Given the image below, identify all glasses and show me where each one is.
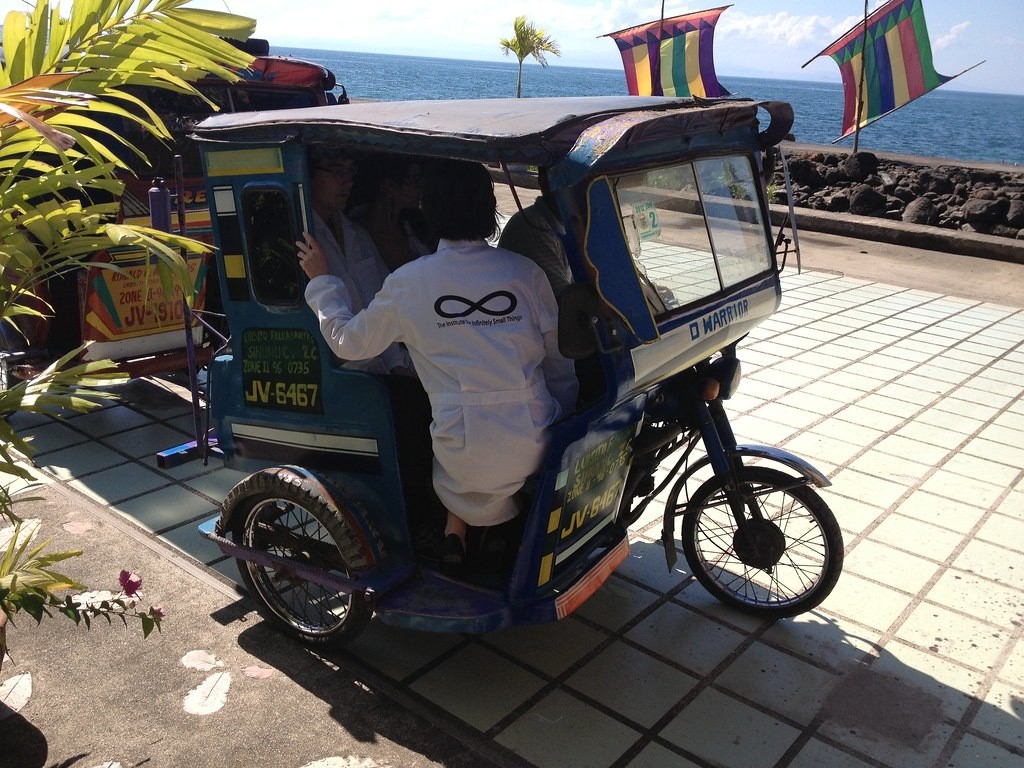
[315,157,360,177]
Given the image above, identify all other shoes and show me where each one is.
[411,521,446,563]
[442,533,471,577]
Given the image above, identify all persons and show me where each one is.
[298,149,612,567]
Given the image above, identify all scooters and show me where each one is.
[151,98,846,654]
[1,35,352,412]
